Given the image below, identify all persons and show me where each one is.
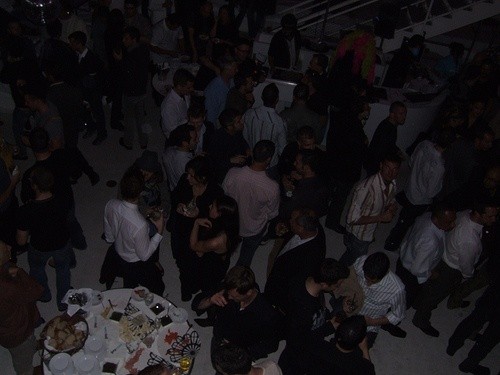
[0,0,500,375]
[191,266,280,375]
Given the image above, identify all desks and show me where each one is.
[43,288,195,375]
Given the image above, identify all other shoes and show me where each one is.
[83,127,96,139]
[447,298,470,308]
[89,171,99,186]
[93,132,106,146]
[446,339,464,355]
[120,137,132,149]
[459,363,490,375]
[413,319,439,337]
[326,221,346,234]
[382,322,406,337]
[114,123,125,131]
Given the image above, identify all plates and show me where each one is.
[42,315,88,356]
